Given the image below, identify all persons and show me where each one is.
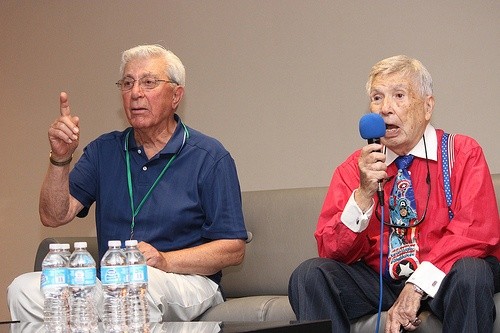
[287,54,500,333]
[5,44,249,322]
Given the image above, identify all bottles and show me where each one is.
[39,244,68,333]
[100,240,127,333]
[61,244,72,260]
[123,239,150,333]
[67,242,97,333]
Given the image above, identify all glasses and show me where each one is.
[116,77,179,90]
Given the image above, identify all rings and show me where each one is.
[411,317,422,327]
[403,322,411,328]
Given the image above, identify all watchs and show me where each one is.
[49,150,73,166]
[413,284,425,296]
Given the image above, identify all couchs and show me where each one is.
[33,173,500,333]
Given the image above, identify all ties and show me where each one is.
[386,155,420,282]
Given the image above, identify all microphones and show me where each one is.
[359,112,386,206]
